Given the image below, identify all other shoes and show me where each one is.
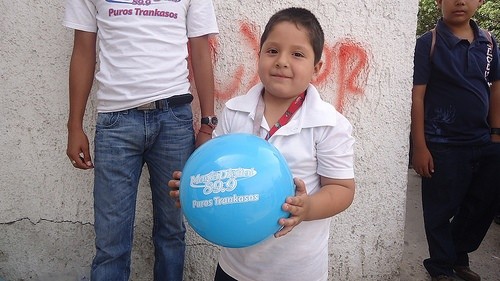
[431,267,481,281]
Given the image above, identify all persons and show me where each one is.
[62,0,220,281]
[411,0,500,281]
[169,7,355,281]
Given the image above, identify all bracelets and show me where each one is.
[199,128,213,134]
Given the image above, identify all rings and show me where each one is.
[71,161,76,164]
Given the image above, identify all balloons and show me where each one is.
[180,133,295,249]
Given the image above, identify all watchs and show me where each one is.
[200,117,218,127]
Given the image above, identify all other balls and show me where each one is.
[177,130,297,248]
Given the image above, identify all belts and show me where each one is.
[136,94,193,111]
